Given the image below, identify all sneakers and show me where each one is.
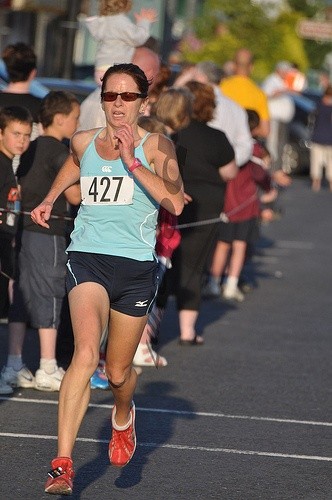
[1,364,35,388]
[107,399,137,467]
[33,367,66,391]
[133,343,168,366]
[44,457,75,496]
[90,367,109,390]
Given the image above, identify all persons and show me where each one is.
[1,0,332,398]
[28,64,190,495]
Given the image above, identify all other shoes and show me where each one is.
[201,285,222,299]
[223,287,244,302]
[0,378,14,393]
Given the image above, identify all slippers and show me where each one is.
[178,334,204,345]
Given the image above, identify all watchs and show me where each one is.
[128,158,141,172]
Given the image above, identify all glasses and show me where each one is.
[102,92,146,101]
